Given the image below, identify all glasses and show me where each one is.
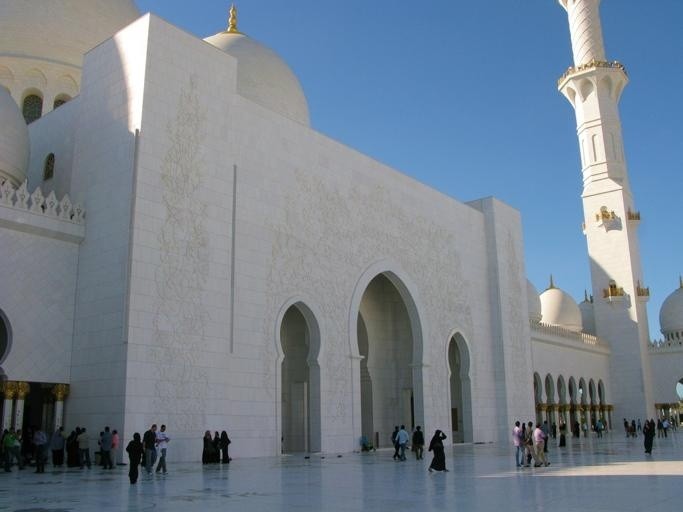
[162,428,166,430]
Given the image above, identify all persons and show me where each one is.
[111,430,119,467]
[552,422,556,437]
[572,419,609,439]
[512,421,525,468]
[524,421,542,468]
[202,429,232,465]
[427,429,450,473]
[533,423,551,467]
[522,423,526,437]
[622,418,641,439]
[392,426,401,460]
[142,424,157,475]
[643,420,655,455]
[97,432,104,447]
[542,421,549,452]
[412,425,425,460]
[101,426,113,470]
[0,425,92,475]
[126,432,144,484]
[394,425,410,461]
[559,419,567,447]
[155,424,170,475]
[656,416,676,439]
